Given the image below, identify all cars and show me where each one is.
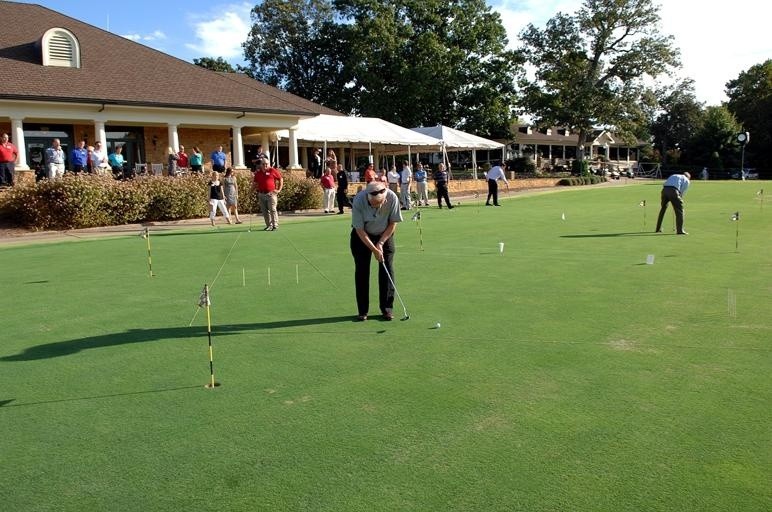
[725,166,760,181]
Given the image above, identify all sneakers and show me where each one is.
[264,225,279,232]
[211,219,244,228]
[485,203,503,207]
[323,208,344,215]
[400,202,454,210]
[676,230,689,235]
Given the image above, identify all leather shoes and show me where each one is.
[383,312,395,321]
[358,314,368,321]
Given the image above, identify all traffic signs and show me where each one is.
[601,142,610,177]
[578,142,586,178]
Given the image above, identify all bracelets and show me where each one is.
[379,241,384,245]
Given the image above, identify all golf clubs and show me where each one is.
[382,260,410,321]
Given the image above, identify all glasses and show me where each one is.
[367,188,386,196]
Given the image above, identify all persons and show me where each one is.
[0,132,20,187]
[702,167,707,180]
[44,136,284,231]
[486,162,509,206]
[655,171,691,234]
[350,182,404,321]
[311,144,454,214]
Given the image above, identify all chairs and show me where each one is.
[134,163,147,173]
[151,163,164,176]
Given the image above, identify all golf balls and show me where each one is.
[436,323,441,327]
